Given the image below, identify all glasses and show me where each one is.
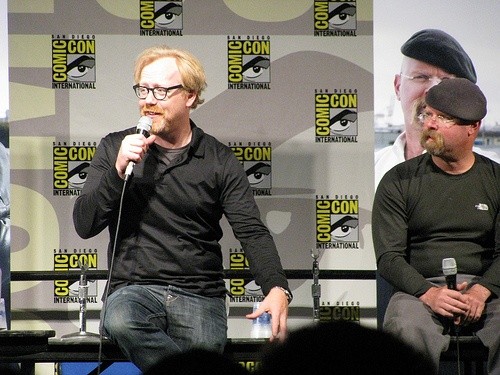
[418,111,459,128]
[133,83,187,100]
[400,72,455,88]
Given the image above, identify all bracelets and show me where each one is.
[275,287,293,303]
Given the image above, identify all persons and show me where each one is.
[72,46,293,373]
[372,77,500,375]
[138,320,437,375]
[371,30,500,192]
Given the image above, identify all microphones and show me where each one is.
[125,116,153,182]
[442,257,460,333]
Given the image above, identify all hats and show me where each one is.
[400,29,477,84]
[424,78,487,122]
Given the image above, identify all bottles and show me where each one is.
[251,303,271,338]
